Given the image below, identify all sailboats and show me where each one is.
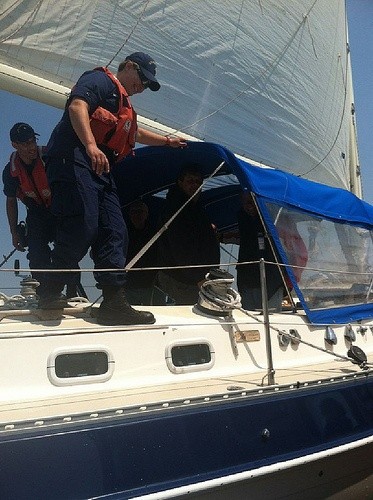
[1,1,373,498]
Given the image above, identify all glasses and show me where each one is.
[11,136,39,147]
[137,69,149,89]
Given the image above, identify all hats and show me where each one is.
[10,122,40,140]
[126,52,160,91]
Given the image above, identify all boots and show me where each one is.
[38,276,67,309]
[67,279,80,303]
[96,287,155,324]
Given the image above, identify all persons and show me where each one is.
[211,192,308,312]
[123,200,163,305]
[157,162,221,306]
[3,124,79,300]
[36,52,187,325]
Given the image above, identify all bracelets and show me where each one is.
[165,136,171,145]
